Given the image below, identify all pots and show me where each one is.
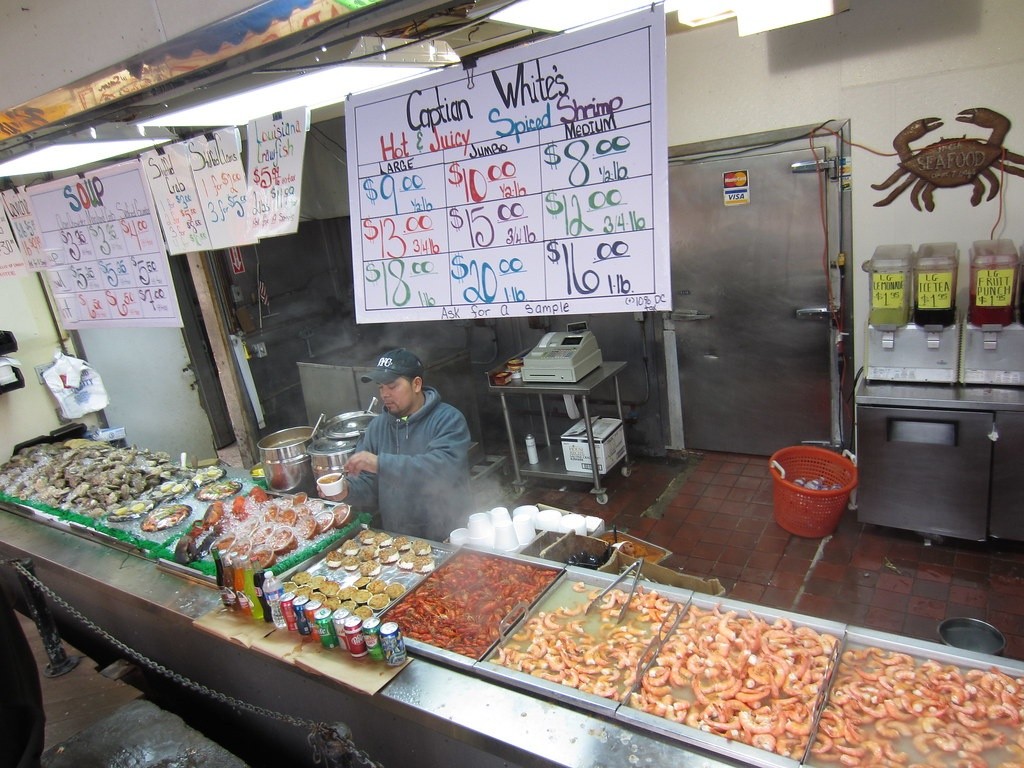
[307,437,359,485]
[257,425,323,498]
[323,410,379,439]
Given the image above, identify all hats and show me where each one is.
[360,348,424,385]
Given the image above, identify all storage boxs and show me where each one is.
[562,414,625,475]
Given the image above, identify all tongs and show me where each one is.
[584,557,644,625]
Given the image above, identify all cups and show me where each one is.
[450,504,587,554]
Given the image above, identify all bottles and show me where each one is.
[249,556,274,622]
[218,549,242,611]
[211,545,231,607]
[261,570,287,628]
[238,554,264,618]
[229,552,251,613]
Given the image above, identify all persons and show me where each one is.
[317,344,473,542]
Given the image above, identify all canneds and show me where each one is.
[305,599,323,641]
[314,607,339,649]
[292,594,311,637]
[333,607,351,650]
[379,622,407,667]
[344,616,368,658]
[362,617,386,661]
[279,592,298,631]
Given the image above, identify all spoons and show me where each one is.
[365,395,378,416]
[337,471,345,482]
[308,412,326,440]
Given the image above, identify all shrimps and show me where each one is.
[487,579,1023,768]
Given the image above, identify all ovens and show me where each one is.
[352,346,487,467]
[296,344,393,431]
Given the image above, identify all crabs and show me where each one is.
[871,107,1024,212]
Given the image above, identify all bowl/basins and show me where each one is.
[316,473,344,496]
